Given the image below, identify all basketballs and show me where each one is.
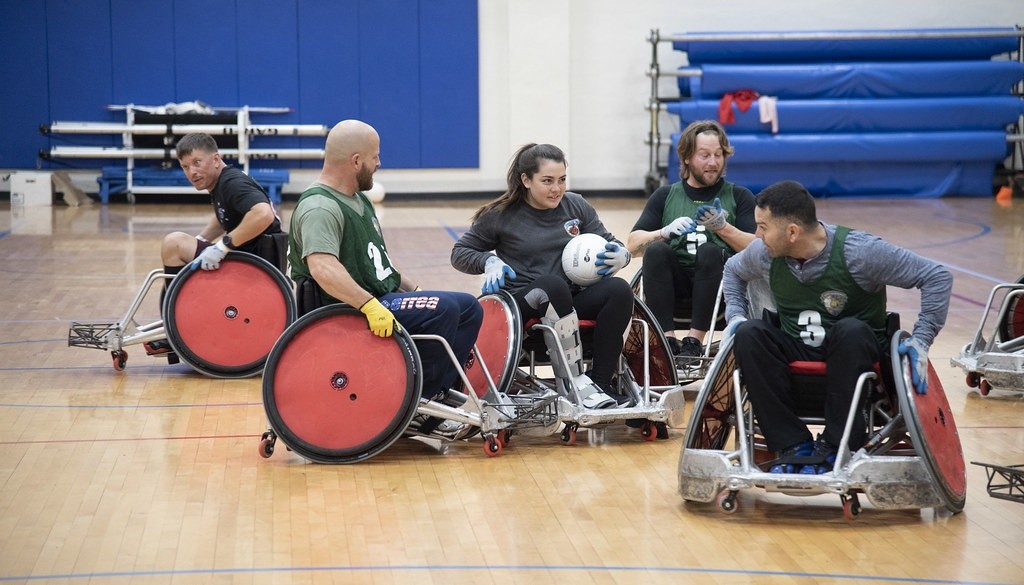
[561,233,612,286]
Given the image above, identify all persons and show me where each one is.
[627,121,756,367]
[723,180,953,472]
[451,144,633,409]
[289,120,483,433]
[142,132,282,355]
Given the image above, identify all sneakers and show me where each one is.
[674,339,702,369]
[766,444,812,472]
[668,336,681,358]
[799,445,835,475]
[409,411,467,436]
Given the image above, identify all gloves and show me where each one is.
[691,198,726,231]
[190,241,225,271]
[898,334,929,394]
[360,299,403,337]
[415,286,423,293]
[597,241,629,278]
[718,311,749,350]
[480,256,517,295]
[661,218,698,241]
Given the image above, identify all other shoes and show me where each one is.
[146,339,173,354]
[598,382,633,409]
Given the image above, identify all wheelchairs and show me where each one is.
[402,288,687,444]
[621,266,728,387]
[675,310,968,522]
[68,228,298,381]
[949,272,1023,397]
[257,274,563,465]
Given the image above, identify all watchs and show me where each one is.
[223,235,237,250]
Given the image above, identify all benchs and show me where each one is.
[97,168,289,205]
